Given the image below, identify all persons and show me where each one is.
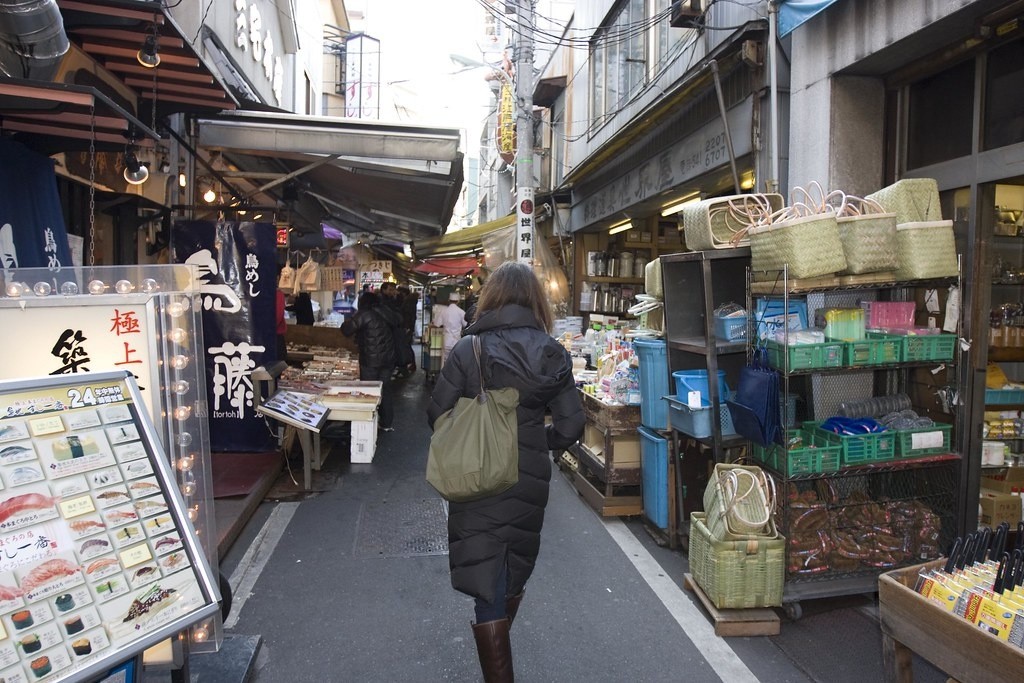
[276,285,288,361]
[424,262,587,683]
[340,282,418,433]
[295,290,314,326]
[294,291,315,326]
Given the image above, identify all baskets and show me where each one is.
[631,258,668,339]
[684,175,961,282]
[688,463,786,610]
[752,330,958,472]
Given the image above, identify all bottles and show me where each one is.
[621,252,633,278]
[604,289,611,311]
[607,255,619,277]
[636,259,648,278]
[629,300,634,308]
[1011,487,1024,522]
[611,294,619,311]
[596,252,606,276]
[988,304,1024,347]
[619,299,625,312]
[593,286,600,311]
[977,503,983,529]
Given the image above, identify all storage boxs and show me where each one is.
[424,327,442,371]
[623,230,652,244]
[657,227,684,247]
[289,380,383,411]
[982,441,1005,465]
[757,301,957,370]
[630,338,668,529]
[584,424,640,469]
[979,467,1024,532]
[350,415,378,463]
[985,389,1024,405]
[714,315,748,343]
[753,418,953,478]
[660,394,800,439]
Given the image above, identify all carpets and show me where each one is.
[186,452,279,497]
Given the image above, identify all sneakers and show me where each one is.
[377,421,394,431]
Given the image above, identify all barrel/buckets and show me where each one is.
[672,369,730,407]
[634,338,668,429]
[637,426,668,528]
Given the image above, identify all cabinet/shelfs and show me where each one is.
[659,246,809,549]
[570,231,654,331]
[744,241,968,620]
[553,388,642,519]
[878,552,1024,682]
[957,234,1023,441]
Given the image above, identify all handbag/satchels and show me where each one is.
[426,387,523,503]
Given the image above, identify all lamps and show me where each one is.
[661,193,706,217]
[252,212,263,220]
[124,153,148,185]
[608,219,637,235]
[137,38,160,66]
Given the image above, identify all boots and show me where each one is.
[470,587,523,683]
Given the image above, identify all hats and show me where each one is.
[449,292,459,301]
